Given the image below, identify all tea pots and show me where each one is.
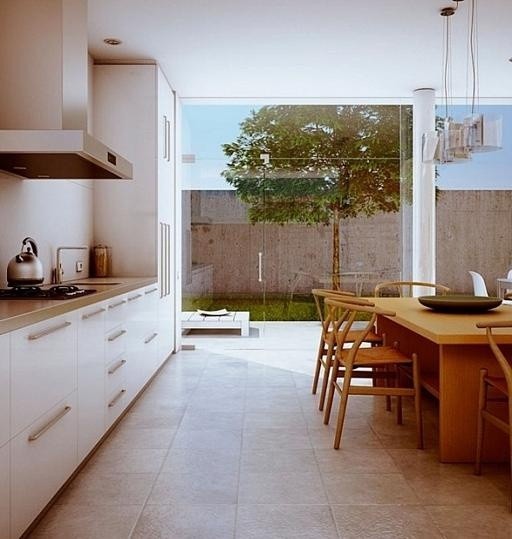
[6,235,45,290]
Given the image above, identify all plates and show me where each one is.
[417,294,503,310]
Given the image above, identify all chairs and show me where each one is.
[283,266,403,318]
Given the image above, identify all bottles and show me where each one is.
[92,244,111,278]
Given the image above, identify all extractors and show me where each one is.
[2,0,134,182]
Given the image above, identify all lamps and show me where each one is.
[420,0,504,166]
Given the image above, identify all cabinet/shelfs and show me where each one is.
[91,64,183,364]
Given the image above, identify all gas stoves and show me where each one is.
[0,286,98,301]
